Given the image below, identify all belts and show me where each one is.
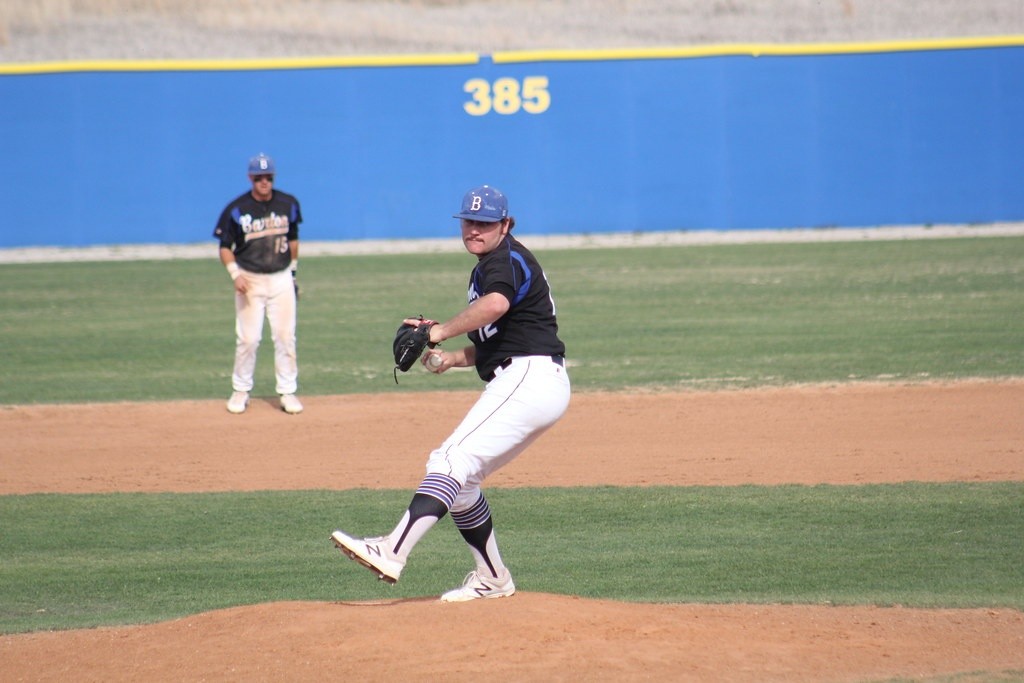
[490,355,566,380]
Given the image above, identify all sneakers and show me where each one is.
[441,568,515,602]
[330,532,404,587]
[227,390,249,414]
[279,393,303,414]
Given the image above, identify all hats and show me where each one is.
[453,185,509,223]
[248,154,275,175]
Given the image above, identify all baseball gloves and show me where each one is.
[393,315,442,384]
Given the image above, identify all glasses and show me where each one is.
[252,177,273,182]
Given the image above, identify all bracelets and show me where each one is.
[291,259,298,271]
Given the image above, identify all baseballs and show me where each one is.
[425,354,442,372]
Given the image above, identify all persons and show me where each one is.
[328,185,571,604]
[212,155,304,415]
